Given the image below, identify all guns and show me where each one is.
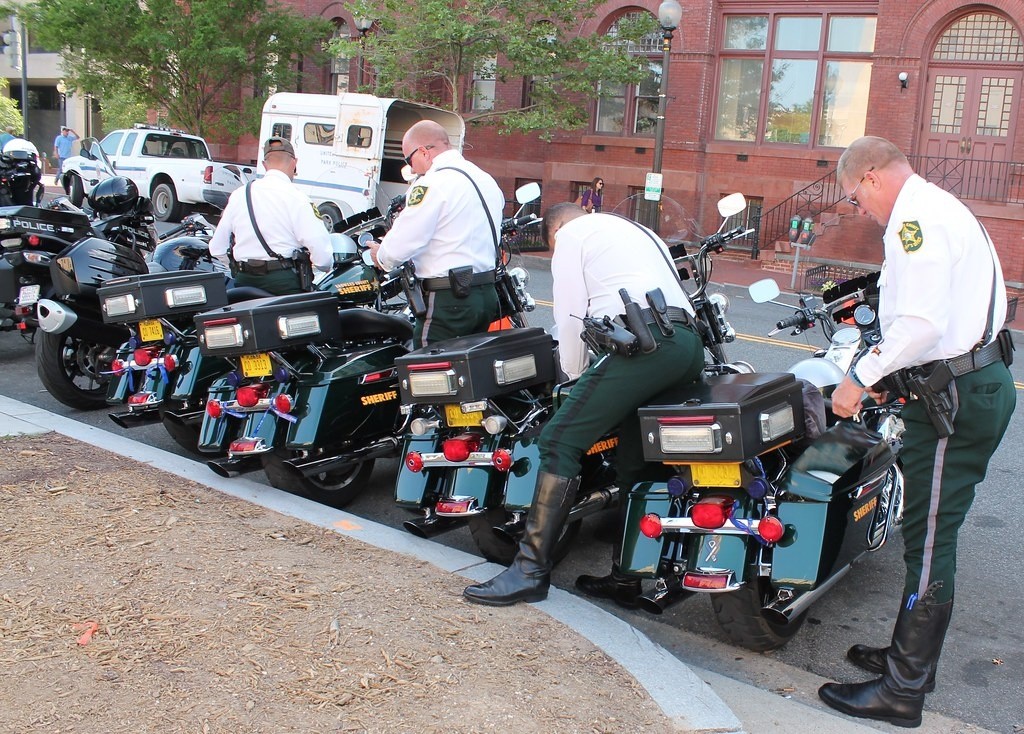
[292,250,313,291]
[906,362,956,438]
[401,260,416,283]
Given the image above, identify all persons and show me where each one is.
[461,202,705,606]
[0,126,16,153]
[581,178,604,215]
[54,126,80,186]
[817,136,1016,728]
[366,119,506,352]
[208,137,334,295]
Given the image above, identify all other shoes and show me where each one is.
[54,177,59,185]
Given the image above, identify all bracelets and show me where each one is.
[849,366,865,388]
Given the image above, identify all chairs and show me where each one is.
[170,147,184,157]
[142,145,147,154]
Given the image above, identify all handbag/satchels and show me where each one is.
[493,270,523,321]
[575,189,593,213]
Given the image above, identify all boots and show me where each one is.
[818,591,953,728]
[464,471,582,607]
[576,493,644,610]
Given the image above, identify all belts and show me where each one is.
[423,271,498,288]
[240,259,292,271]
[908,340,1002,375]
[643,306,699,330]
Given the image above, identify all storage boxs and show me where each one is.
[394,326,556,404]
[48,237,149,298]
[192,291,343,356]
[96,270,228,325]
[286,344,408,449]
[0,205,96,243]
[638,372,806,462]
[772,422,896,589]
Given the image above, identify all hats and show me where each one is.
[263,137,295,157]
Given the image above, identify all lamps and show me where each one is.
[899,71,909,93]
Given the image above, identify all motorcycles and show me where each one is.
[0,134,909,653]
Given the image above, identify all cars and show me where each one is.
[0,133,42,204]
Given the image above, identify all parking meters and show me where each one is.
[786,215,812,290]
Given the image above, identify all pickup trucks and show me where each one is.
[61,122,256,224]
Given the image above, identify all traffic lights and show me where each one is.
[1,16,23,72]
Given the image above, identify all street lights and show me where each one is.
[646,1,684,226]
[56,79,70,128]
[352,8,375,91]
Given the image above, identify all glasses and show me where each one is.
[597,182,603,185]
[404,145,434,167]
[845,166,877,206]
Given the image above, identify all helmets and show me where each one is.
[88,177,139,212]
[786,358,847,400]
